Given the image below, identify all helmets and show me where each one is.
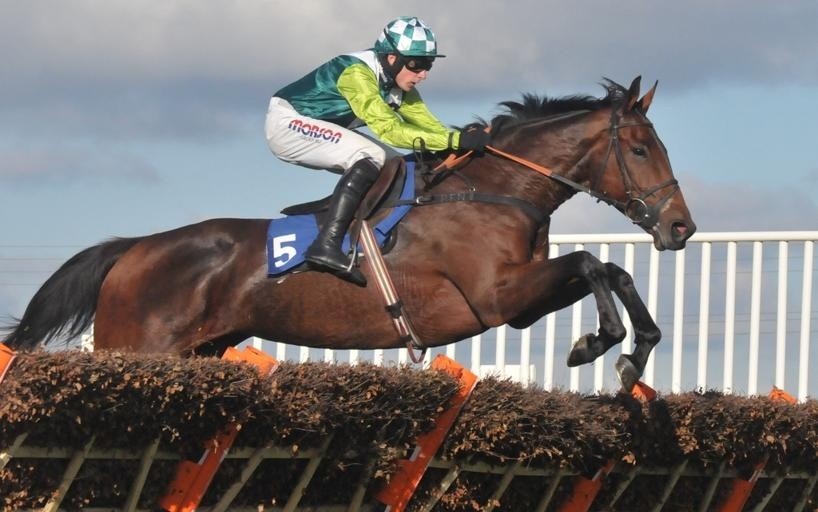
[374,17,447,58]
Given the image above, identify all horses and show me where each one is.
[1,74,697,396]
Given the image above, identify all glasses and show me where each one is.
[402,56,433,72]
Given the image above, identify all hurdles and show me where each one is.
[0,344,813,511]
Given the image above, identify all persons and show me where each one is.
[266,16,492,286]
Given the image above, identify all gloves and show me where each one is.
[459,131,489,154]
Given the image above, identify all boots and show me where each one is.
[304,159,380,286]
